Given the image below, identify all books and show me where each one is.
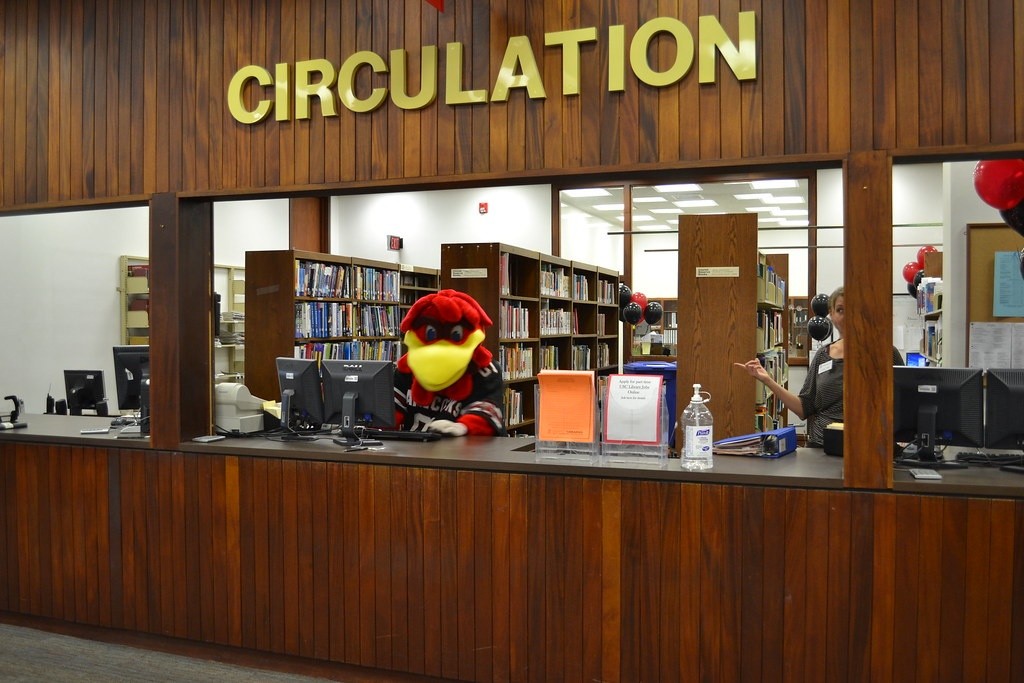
[215,309,245,378]
[755,261,788,436]
[541,262,588,301]
[295,258,438,363]
[598,313,606,336]
[499,299,570,340]
[574,309,579,335]
[505,375,609,442]
[916,276,943,367]
[633,312,678,354]
[599,280,615,305]
[500,251,511,296]
[711,427,797,457]
[498,342,610,381]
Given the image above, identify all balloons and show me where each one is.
[807,293,832,341]
[619,283,663,329]
[973,158,1024,281]
[902,245,938,299]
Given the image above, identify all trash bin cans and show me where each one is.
[624,361,678,450]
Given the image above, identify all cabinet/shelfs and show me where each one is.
[788,295,809,367]
[632,298,677,355]
[120,252,246,387]
[679,211,790,435]
[918,309,943,363]
[441,241,620,435]
[245,249,439,397]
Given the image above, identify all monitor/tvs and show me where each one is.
[63,368,105,409]
[275,357,324,441]
[112,344,150,438]
[892,366,984,470]
[985,368,1024,474]
[321,359,395,446]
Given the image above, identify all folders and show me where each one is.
[712,428,797,458]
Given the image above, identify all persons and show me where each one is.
[733,287,905,448]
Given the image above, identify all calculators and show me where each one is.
[909,468,943,479]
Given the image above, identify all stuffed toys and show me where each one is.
[389,288,504,438]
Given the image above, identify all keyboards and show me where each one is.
[338,427,441,442]
[955,452,1024,466]
